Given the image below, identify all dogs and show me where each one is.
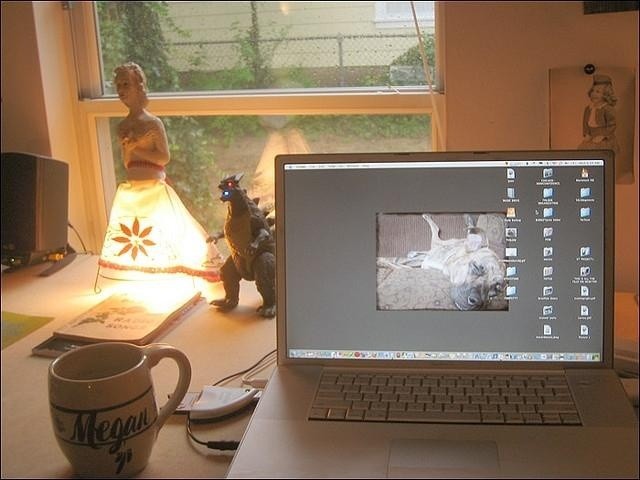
[376,213,507,310]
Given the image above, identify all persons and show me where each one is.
[578,73,618,153]
[111,61,171,181]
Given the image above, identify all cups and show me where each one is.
[48,341,192,480]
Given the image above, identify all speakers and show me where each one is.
[0,150,68,268]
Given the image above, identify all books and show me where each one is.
[31,295,208,360]
[53,286,203,347]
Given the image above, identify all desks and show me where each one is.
[1,247,640,479]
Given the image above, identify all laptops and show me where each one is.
[227,150,639,479]
